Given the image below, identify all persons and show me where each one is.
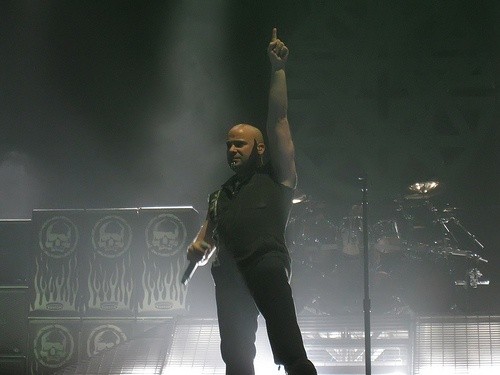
[186,28,317,375]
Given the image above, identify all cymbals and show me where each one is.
[402,181,446,200]
[435,202,462,210]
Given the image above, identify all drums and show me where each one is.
[372,220,405,254]
[339,215,370,256]
[285,216,336,252]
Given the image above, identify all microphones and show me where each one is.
[181,242,208,285]
